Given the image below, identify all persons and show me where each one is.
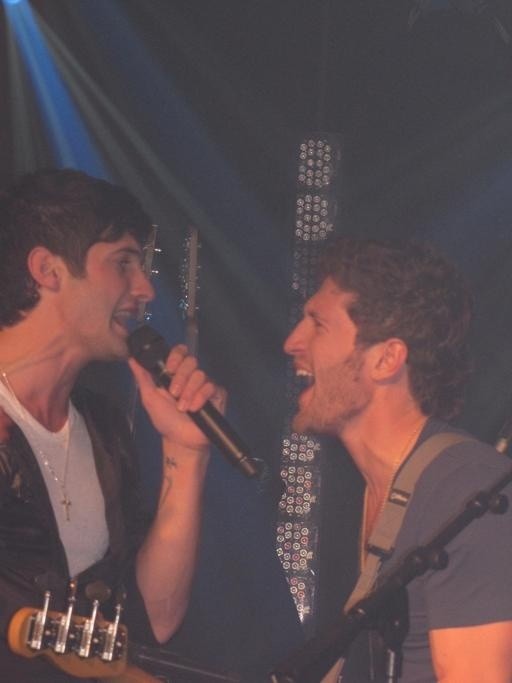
[283,235,512,683]
[0,165,229,683]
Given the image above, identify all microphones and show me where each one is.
[126,323,262,481]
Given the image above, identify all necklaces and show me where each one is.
[361,416,427,574]
[0,368,72,521]
